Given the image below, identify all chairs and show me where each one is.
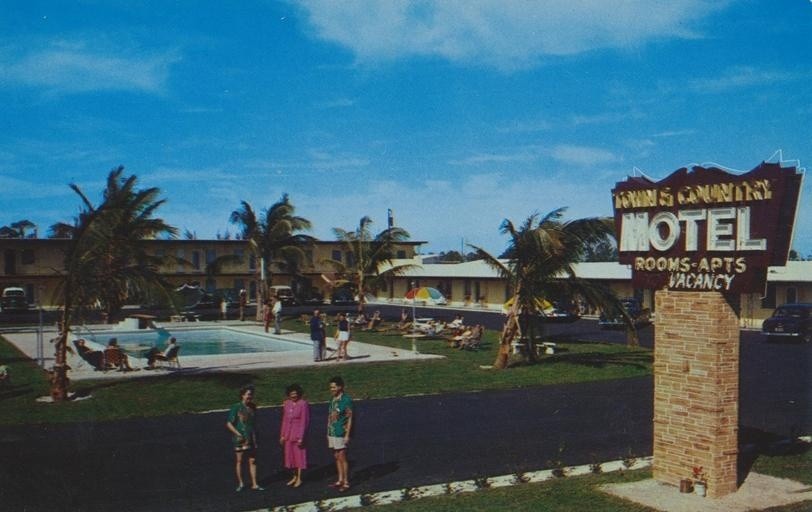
[297,307,487,353]
[68,335,182,378]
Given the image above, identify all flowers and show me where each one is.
[688,464,706,482]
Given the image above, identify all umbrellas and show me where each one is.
[504,293,551,313]
[403,286,445,305]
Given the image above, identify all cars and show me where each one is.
[760,302,811,343]
[331,288,355,305]
[299,287,323,305]
[544,303,580,322]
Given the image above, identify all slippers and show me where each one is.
[235,483,246,494]
[251,485,267,492]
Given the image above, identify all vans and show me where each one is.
[269,285,296,307]
[214,288,241,309]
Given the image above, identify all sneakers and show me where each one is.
[327,481,343,489]
[337,483,354,493]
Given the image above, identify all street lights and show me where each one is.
[409,280,418,353]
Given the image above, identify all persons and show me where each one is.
[271,295,283,334]
[145,336,176,368]
[279,384,310,488]
[307,308,484,361]
[220,298,227,319]
[326,378,353,491]
[262,299,271,333]
[109,337,133,369]
[240,290,246,322]
[227,385,266,493]
[77,337,106,367]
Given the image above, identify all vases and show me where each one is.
[693,481,706,496]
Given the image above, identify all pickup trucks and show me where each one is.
[0,287,29,313]
[599,297,649,331]
